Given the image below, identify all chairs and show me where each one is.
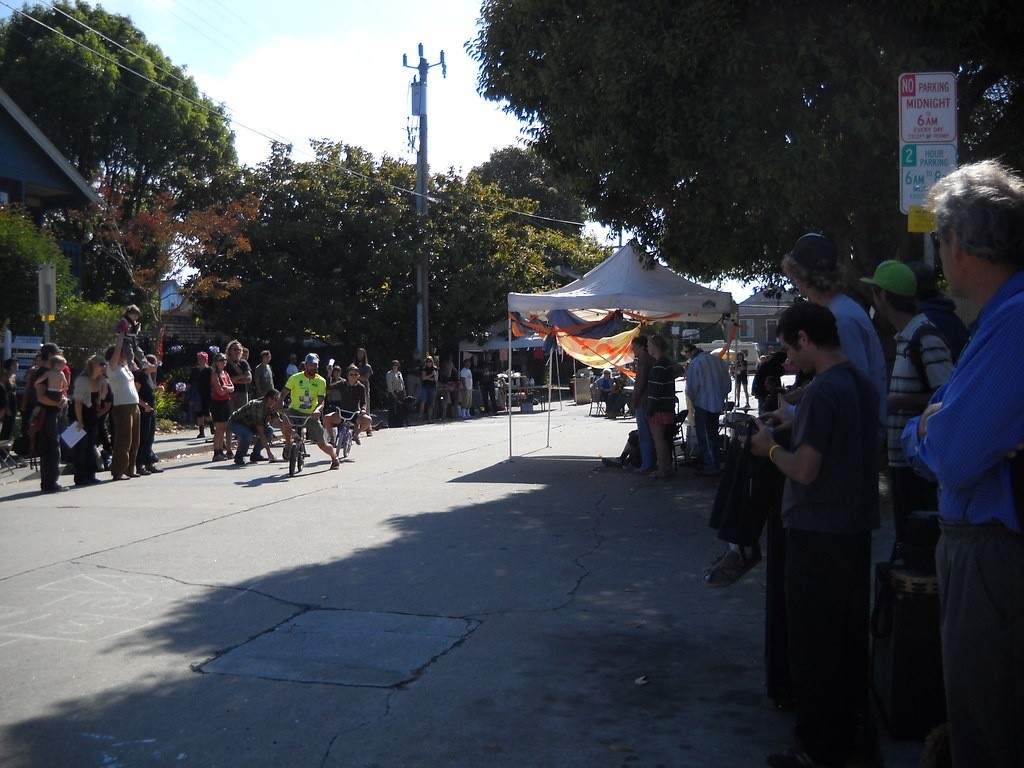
[0,421,18,475]
[718,402,735,435]
[589,385,606,416]
[670,410,690,469]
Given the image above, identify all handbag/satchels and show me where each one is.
[900,510,941,576]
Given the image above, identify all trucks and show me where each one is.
[697,340,759,373]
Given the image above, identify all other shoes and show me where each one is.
[331,459,340,470]
[282,444,292,460]
[634,468,654,474]
[328,439,334,444]
[649,469,673,479]
[702,541,762,587]
[601,458,623,469]
[129,364,156,371]
[353,436,361,445]
[196,431,268,464]
[695,470,720,476]
[41,466,164,490]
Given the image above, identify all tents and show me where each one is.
[458,317,561,404]
[506,239,739,464]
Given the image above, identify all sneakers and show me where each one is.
[767,744,830,768]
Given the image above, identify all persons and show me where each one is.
[592,233,975,586]
[899,158,1024,767]
[0,305,498,492]
[737,300,886,767]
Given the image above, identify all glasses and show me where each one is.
[929,231,945,249]
[350,373,358,377]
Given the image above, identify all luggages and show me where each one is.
[870,563,948,740]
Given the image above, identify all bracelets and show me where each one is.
[769,445,782,463]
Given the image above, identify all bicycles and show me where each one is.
[329,404,367,459]
[274,408,319,477]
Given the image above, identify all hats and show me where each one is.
[90,354,107,364]
[305,353,320,364]
[791,233,839,269]
[41,343,64,355]
[859,260,917,296]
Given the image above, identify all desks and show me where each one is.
[622,386,635,417]
[502,384,552,413]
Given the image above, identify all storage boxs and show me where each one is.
[520,399,533,413]
